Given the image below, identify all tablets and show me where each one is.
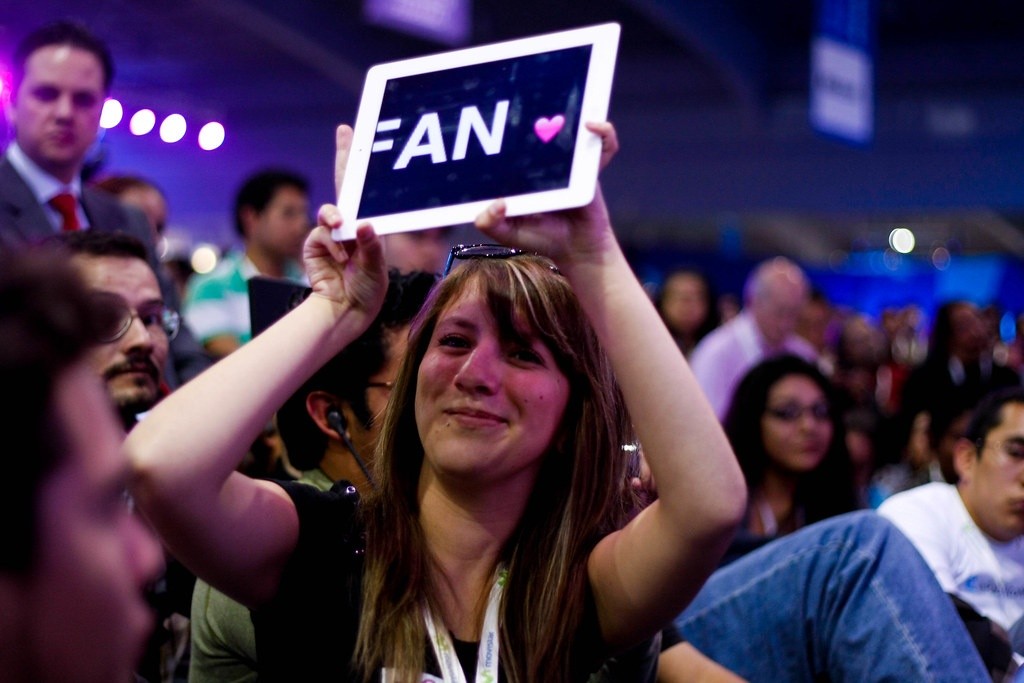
[328,23,620,241]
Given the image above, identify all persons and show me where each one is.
[113,123,752,683]
[637,253,1024,683]
[0,20,178,391]
[0,168,446,683]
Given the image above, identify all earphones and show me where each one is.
[326,407,347,434]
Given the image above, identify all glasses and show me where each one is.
[96,303,179,343]
[442,244,562,275]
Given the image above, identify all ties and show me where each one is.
[48,194,81,233]
